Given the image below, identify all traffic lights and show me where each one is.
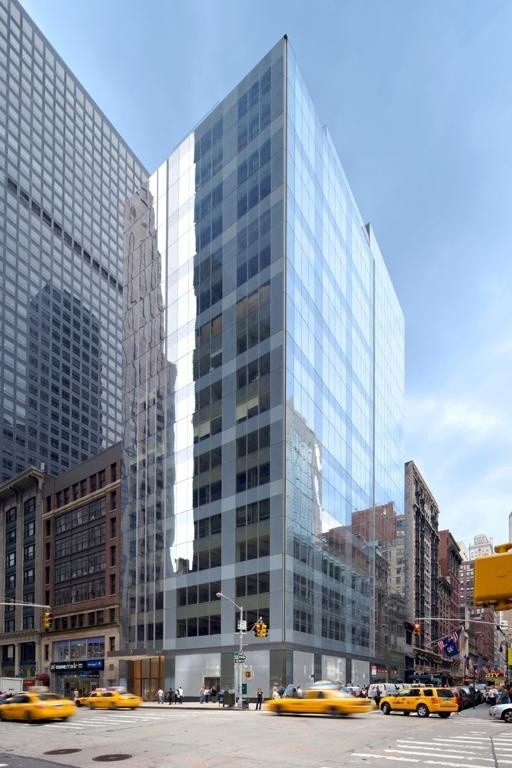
[254,624,260,636]
[414,624,419,637]
[262,624,268,638]
[45,612,53,628]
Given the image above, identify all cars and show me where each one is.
[489,704,512,722]
[262,680,376,718]
[86,687,142,710]
[76,687,108,707]
[1,691,21,700]
[448,683,497,710]
[1,686,77,723]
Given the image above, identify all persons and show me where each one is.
[72,688,80,697]
[154,684,381,710]
[488,684,499,706]
[499,685,509,704]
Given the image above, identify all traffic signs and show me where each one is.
[235,658,245,663]
[239,655,247,658]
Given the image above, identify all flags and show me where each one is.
[436,627,463,657]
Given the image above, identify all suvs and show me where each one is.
[379,687,459,717]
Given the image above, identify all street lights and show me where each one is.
[499,640,508,679]
[216,592,244,709]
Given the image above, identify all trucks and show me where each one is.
[0,676,23,693]
[368,682,433,704]
